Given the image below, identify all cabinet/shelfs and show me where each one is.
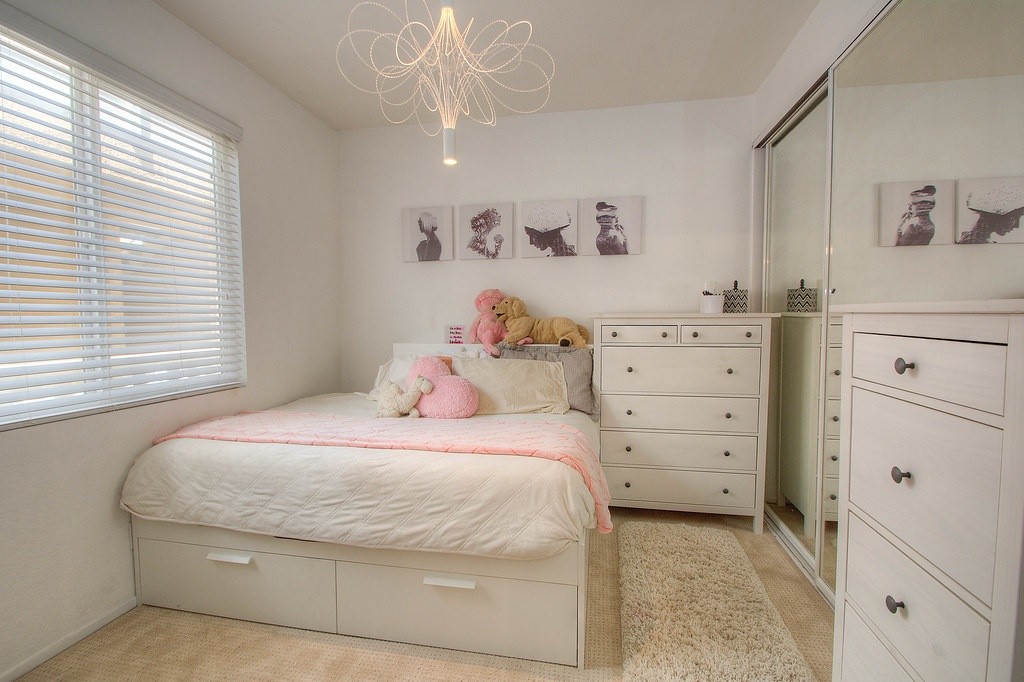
[828,298,1024,682]
[587,311,782,537]
[780,276,843,540]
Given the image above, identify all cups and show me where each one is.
[703,296,721,314]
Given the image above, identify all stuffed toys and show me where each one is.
[468,288,588,358]
[377,354,478,419]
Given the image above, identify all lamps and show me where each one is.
[335,0,556,165]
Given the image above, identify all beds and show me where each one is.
[118,343,614,672]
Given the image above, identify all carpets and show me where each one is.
[617,519,818,682]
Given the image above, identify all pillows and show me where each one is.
[498,342,600,423]
[375,350,480,391]
[450,354,570,415]
